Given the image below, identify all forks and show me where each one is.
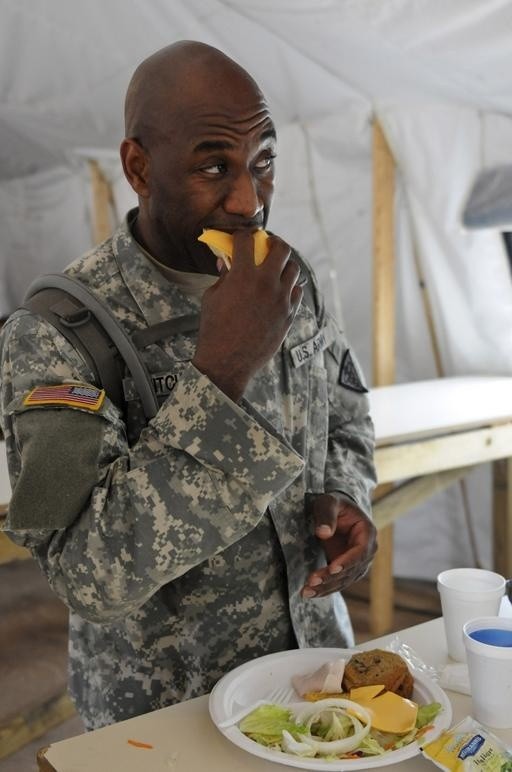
[218,685,295,732]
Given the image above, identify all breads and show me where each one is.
[344,648,408,694]
[342,671,414,702]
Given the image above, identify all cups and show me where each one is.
[434,568,512,732]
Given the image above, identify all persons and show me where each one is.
[0,38,380,734]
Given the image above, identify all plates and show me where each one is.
[208,648,453,772]
